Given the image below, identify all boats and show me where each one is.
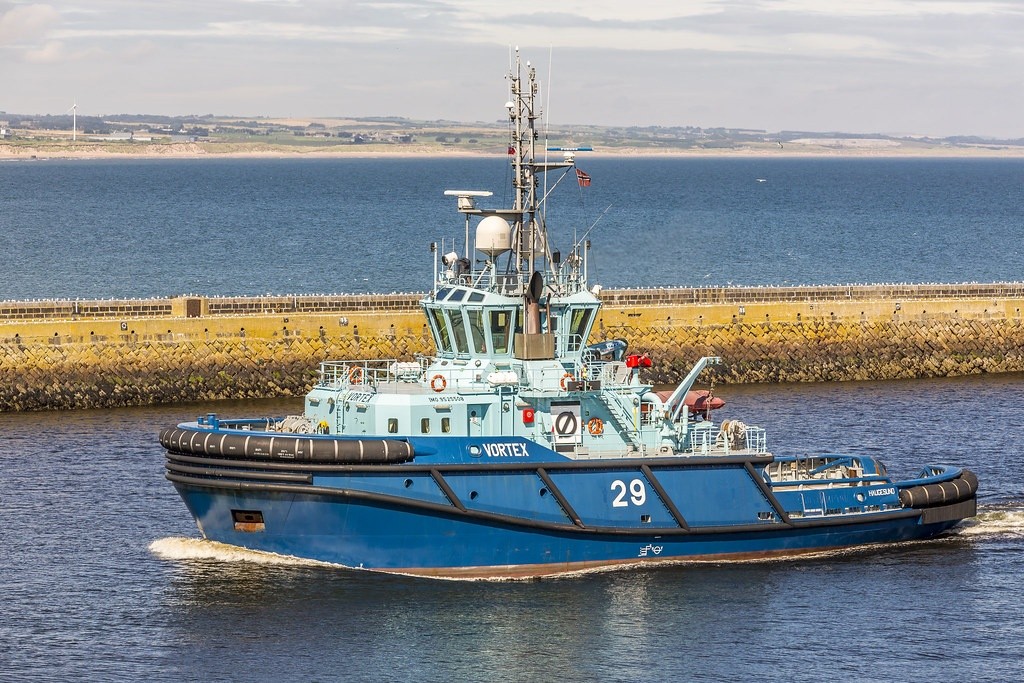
[161,43,979,580]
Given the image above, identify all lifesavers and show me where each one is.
[560,372,576,392]
[431,373,446,393]
[348,367,364,386]
[587,416,605,437]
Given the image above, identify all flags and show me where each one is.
[576,167,591,187]
